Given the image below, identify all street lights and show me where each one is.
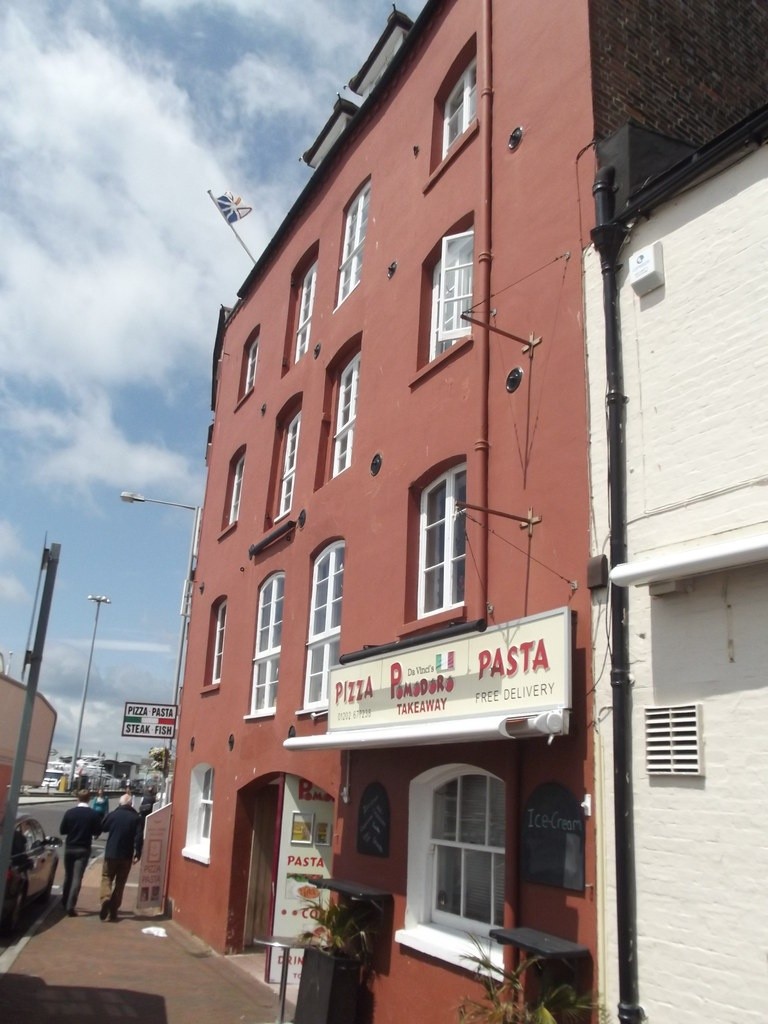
[120,490,202,789]
[66,596,113,792]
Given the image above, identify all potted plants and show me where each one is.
[291,891,383,1024]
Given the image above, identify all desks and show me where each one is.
[254,935,310,1024]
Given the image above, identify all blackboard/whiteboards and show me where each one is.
[519,782,592,891]
[355,781,391,855]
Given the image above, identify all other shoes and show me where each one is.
[66,909,78,917]
[99,899,111,921]
[109,909,117,922]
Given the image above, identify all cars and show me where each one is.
[0,813,59,936]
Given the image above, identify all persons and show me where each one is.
[120,787,135,807]
[141,787,155,812]
[91,789,109,839]
[99,795,144,922]
[59,789,102,916]
